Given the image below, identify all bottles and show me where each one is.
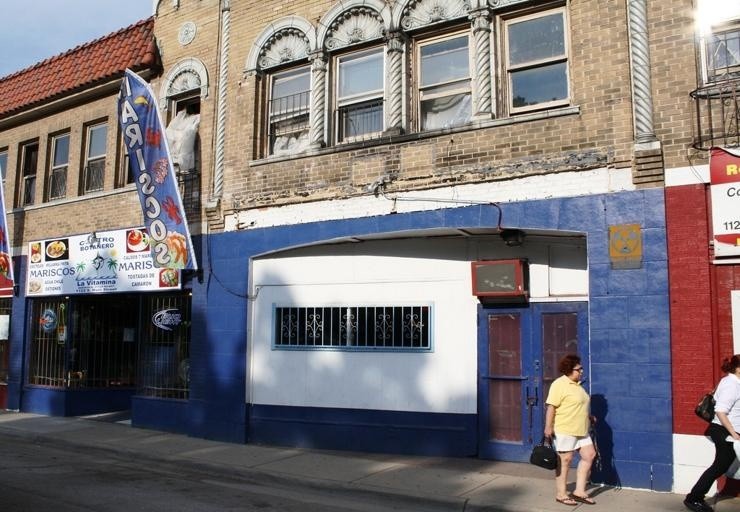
[57,308,66,344]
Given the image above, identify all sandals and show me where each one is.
[571,490,595,505]
[556,497,577,506]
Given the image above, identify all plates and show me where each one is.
[127,232,150,252]
[29,280,43,293]
[31,253,41,263]
[46,241,67,258]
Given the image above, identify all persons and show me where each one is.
[543,352,598,506]
[682,353,740,512]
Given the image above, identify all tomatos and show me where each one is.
[129,232,141,245]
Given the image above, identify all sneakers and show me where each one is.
[683,494,715,512]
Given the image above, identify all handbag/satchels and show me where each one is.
[695,393,717,422]
[529,436,559,470]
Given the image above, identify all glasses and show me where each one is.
[573,367,583,372]
